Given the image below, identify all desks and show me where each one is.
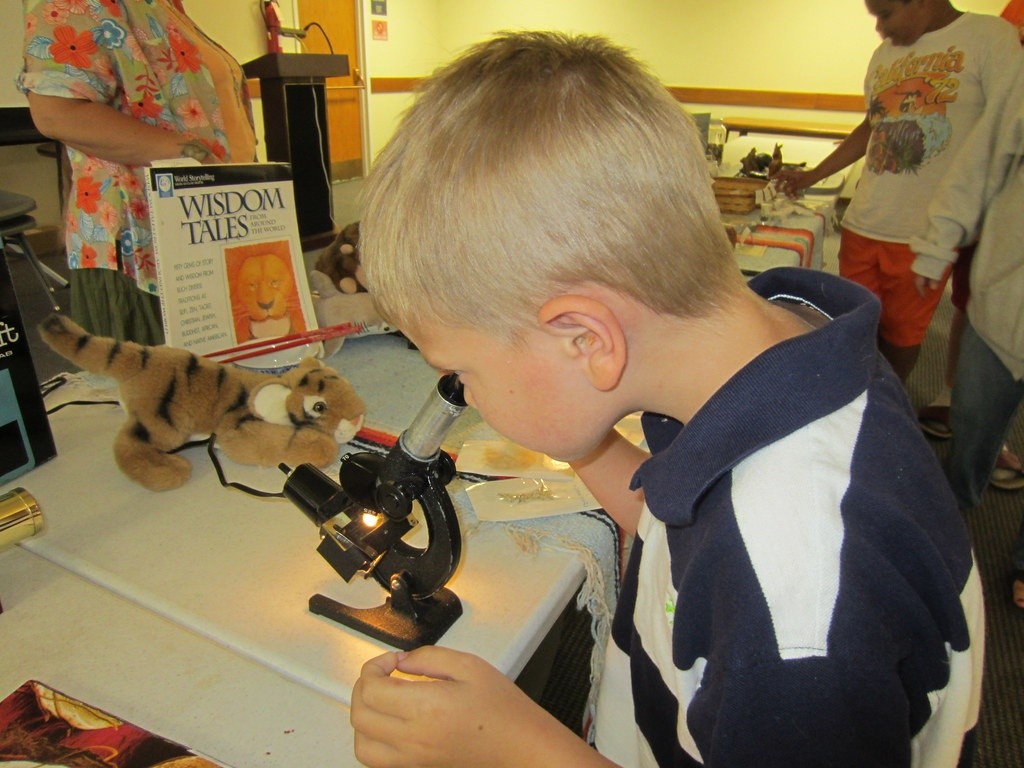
[0,203,833,709]
[0,542,369,768]
[720,116,859,144]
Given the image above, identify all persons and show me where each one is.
[16,0,257,347]
[349,31,985,768]
[768,0,1024,525]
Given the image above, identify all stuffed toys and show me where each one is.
[37,311,367,492]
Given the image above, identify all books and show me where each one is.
[145,162,320,364]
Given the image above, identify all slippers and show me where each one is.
[990,446,1024,489]
[918,404,955,437]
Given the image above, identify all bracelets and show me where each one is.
[180,138,210,163]
